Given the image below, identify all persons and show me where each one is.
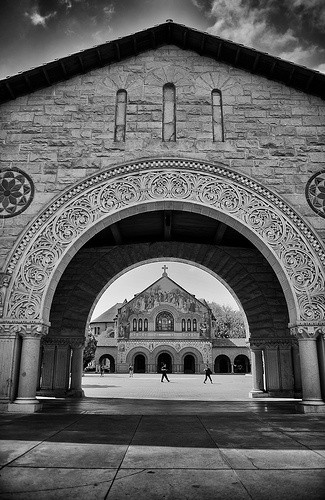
[129,366,134,377]
[203,365,213,384]
[160,363,170,382]
[100,366,104,377]
